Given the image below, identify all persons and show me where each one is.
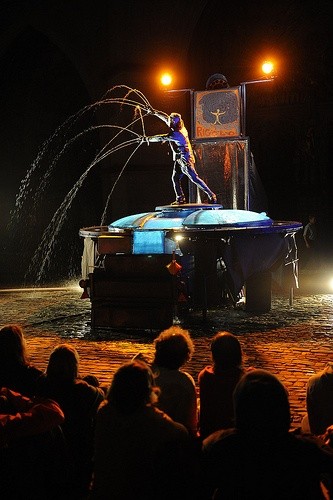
[200,336,244,445]
[201,368,333,500]
[38,345,106,499]
[0,325,46,500]
[139,110,219,206]
[148,327,198,441]
[0,384,65,500]
[81,361,193,500]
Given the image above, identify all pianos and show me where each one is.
[91,253,195,331]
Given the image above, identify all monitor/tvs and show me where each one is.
[130,230,165,255]
